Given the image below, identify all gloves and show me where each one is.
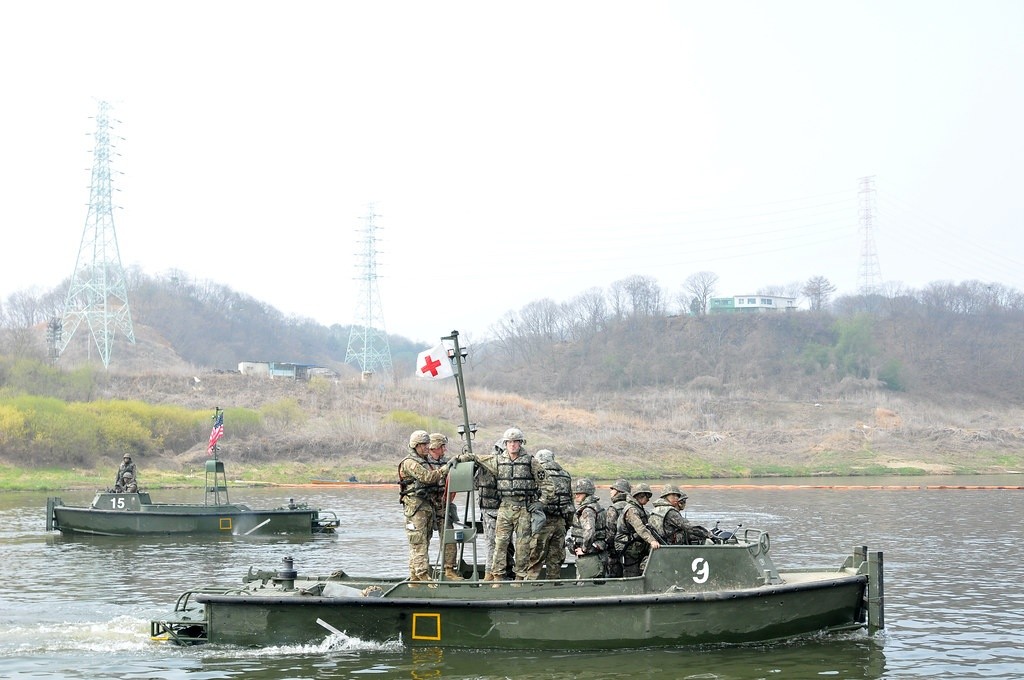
[527,501,544,513]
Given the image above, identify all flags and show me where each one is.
[207,412,223,456]
[416,344,454,380]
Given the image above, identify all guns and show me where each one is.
[647,525,668,545]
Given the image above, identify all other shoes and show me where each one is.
[492,575,503,588]
[420,574,438,588]
[408,577,420,588]
[510,576,523,586]
[483,572,493,581]
[444,569,465,580]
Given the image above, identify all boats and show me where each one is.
[42,404,342,538]
[147,328,887,658]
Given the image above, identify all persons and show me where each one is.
[524,449,574,586]
[446,428,555,588]
[567,479,612,586]
[398,430,465,587]
[117,472,139,493]
[606,478,714,578]
[478,439,515,581]
[116,453,137,486]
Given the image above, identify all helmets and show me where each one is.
[659,484,681,497]
[410,430,430,448]
[124,452,131,458]
[572,478,596,495]
[534,449,554,463]
[504,427,523,441]
[494,438,507,452]
[677,490,688,502]
[429,433,448,449]
[631,483,652,497]
[610,479,632,492]
[123,472,132,478]
